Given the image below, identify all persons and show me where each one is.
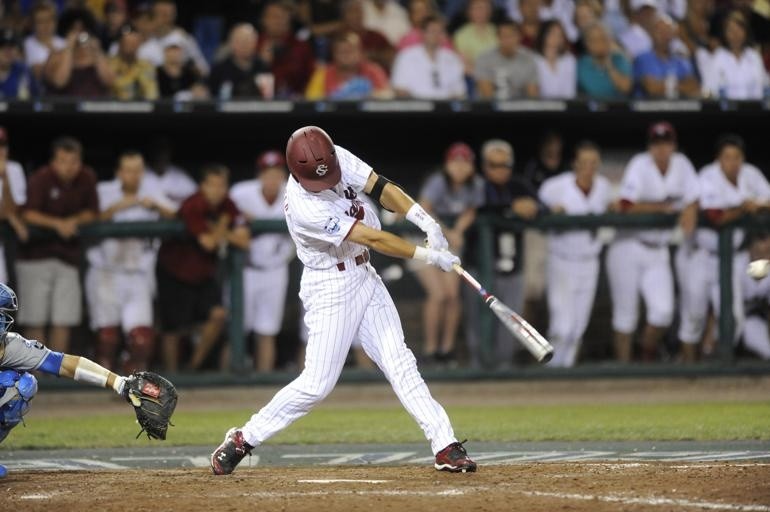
[0,285,178,477]
[1,1,409,371]
[387,2,768,373]
[210,123,477,475]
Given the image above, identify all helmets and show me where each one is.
[286,125,341,193]
[0,282,19,343]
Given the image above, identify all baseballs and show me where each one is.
[747,260,769,279]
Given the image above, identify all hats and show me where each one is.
[483,139,512,169]
[260,152,284,169]
[648,121,676,142]
[447,145,473,162]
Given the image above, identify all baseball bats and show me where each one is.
[453,264,554,363]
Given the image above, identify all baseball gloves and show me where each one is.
[124,372,177,440]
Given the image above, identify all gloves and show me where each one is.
[429,250,462,273]
[427,224,449,250]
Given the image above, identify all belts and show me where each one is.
[337,249,369,271]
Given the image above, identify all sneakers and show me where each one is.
[434,439,476,472]
[210,426,255,475]
